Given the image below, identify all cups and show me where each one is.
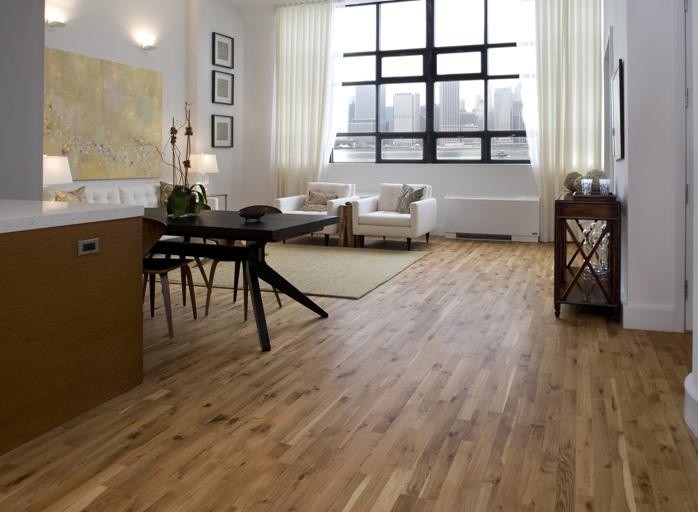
[579,177,593,197]
[598,179,610,195]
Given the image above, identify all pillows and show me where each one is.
[159,181,175,210]
[54,185,87,204]
[299,189,337,212]
[396,183,424,213]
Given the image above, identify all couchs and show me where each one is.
[43,178,218,267]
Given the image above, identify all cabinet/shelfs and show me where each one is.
[554,190,622,324]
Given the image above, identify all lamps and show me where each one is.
[140,40,156,52]
[46,18,66,28]
[42,155,73,186]
[187,153,219,210]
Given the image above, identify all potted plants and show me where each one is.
[167,183,208,217]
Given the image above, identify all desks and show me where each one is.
[144,207,340,352]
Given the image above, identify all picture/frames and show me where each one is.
[610,58,624,161]
[212,115,233,148]
[212,70,234,105]
[212,32,234,69]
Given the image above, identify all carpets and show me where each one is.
[141,242,433,299]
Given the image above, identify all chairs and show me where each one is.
[274,181,357,246]
[205,205,282,320]
[142,205,215,337]
[344,183,437,251]
